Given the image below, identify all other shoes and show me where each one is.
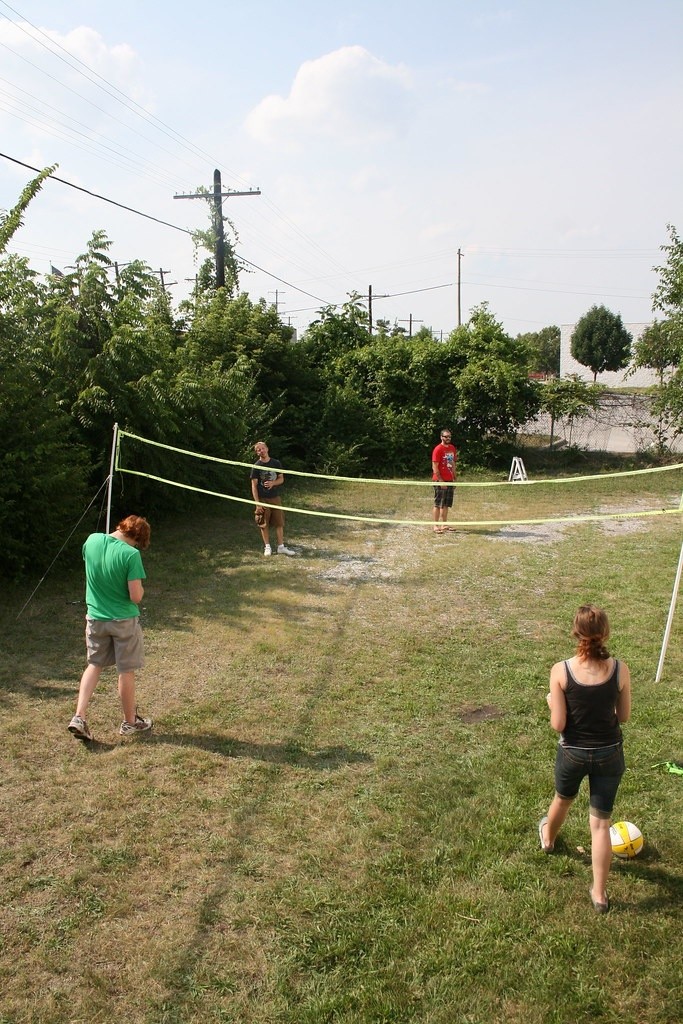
[591,884,608,913]
[441,526,456,531]
[538,817,555,850]
[434,528,442,534]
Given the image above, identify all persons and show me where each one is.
[432,429,457,533]
[68,515,153,741]
[250,442,295,556]
[539,603,631,910]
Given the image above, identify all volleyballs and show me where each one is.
[608,820,644,859]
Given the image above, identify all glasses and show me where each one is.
[441,435,452,438]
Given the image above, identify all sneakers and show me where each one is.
[263,544,272,556]
[277,546,295,556]
[119,714,152,735]
[68,716,92,742]
